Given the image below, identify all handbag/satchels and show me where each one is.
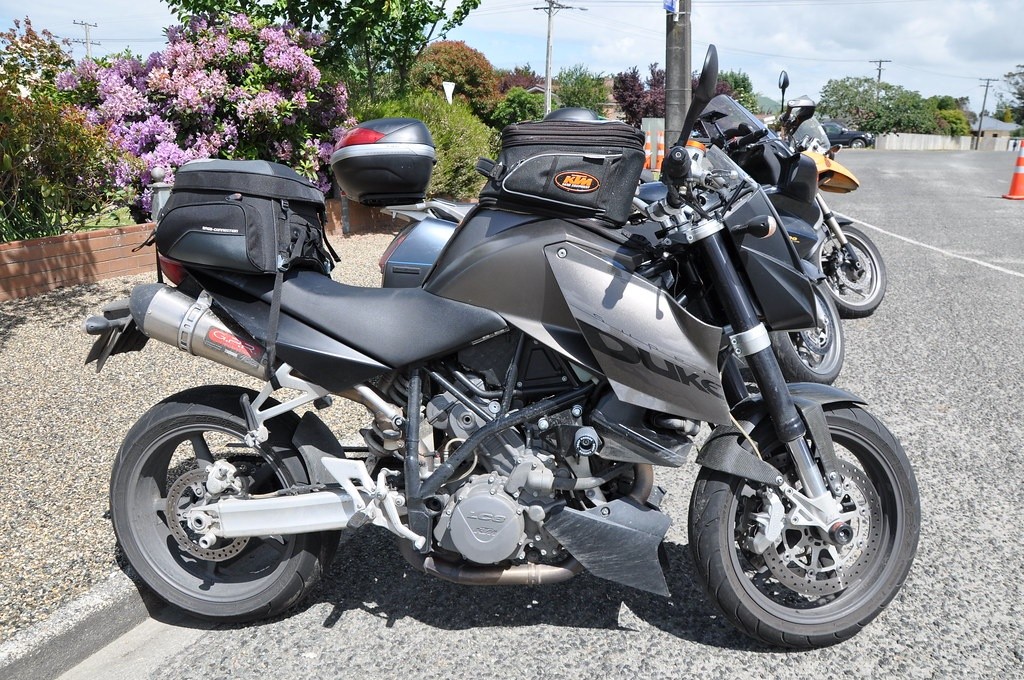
[478,118,642,227]
[155,160,330,273]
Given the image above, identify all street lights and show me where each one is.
[544,6,590,117]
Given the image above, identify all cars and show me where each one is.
[819,121,875,149]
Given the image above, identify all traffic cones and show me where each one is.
[1002,140,1024,200]
[644,131,652,171]
[652,130,664,171]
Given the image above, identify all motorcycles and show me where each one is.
[78,42,922,653]
[543,68,887,318]
[329,71,850,386]
